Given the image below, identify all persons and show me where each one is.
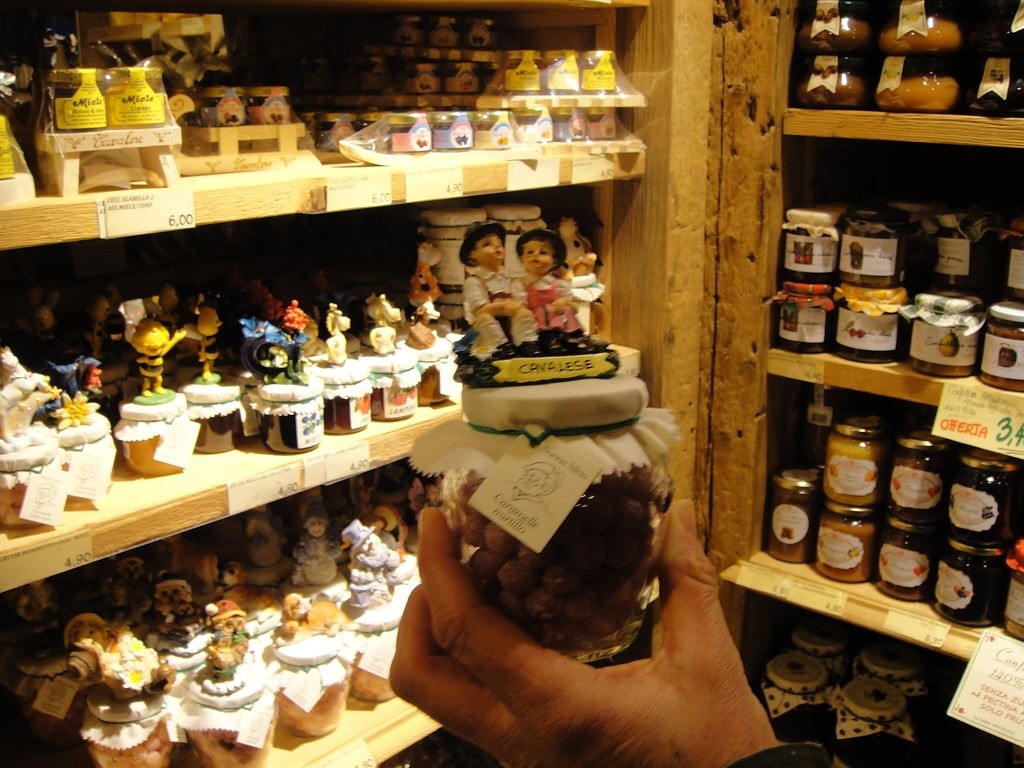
[513,226,589,341]
[456,216,543,359]
[2,468,446,707]
[387,499,847,767]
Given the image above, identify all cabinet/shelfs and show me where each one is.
[1,1,1024,766]
